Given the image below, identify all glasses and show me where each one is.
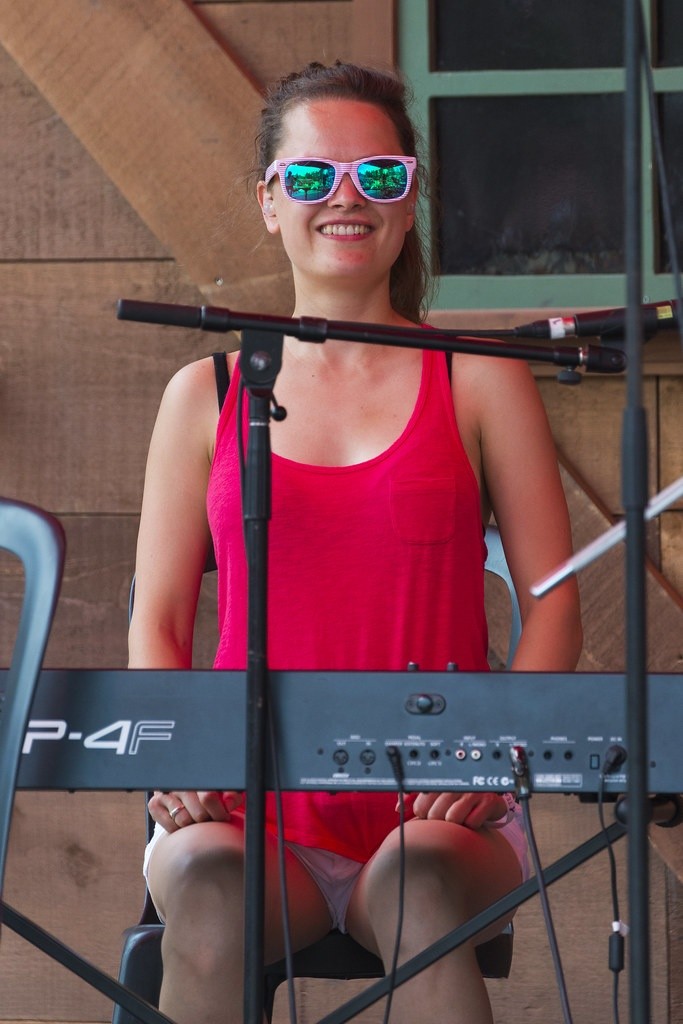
[265,155,417,204]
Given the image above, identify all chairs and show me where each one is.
[113,523,520,1024]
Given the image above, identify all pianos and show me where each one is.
[1,662,683,1023]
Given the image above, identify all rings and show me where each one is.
[170,805,185,818]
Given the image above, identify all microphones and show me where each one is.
[512,296,683,340]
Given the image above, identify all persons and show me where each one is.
[127,60,582,1024]
